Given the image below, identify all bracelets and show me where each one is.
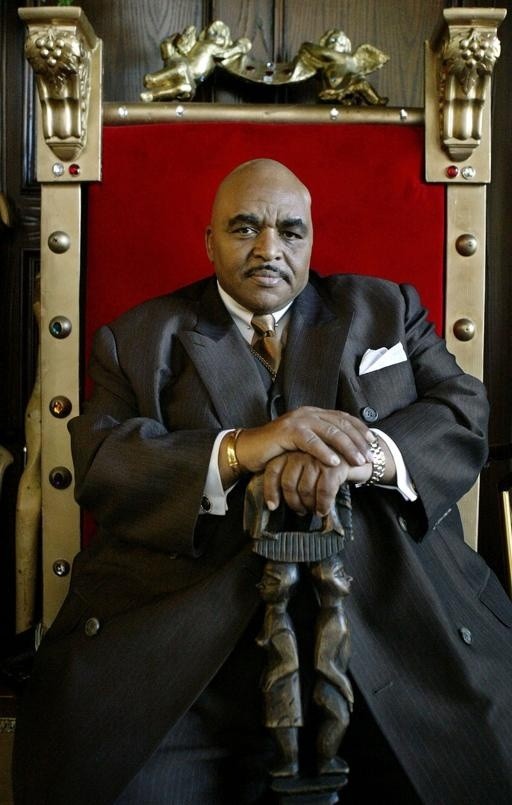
[225,427,242,477]
[352,438,387,489]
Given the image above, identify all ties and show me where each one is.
[248,314,285,384]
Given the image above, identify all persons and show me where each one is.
[8,157,511,804]
